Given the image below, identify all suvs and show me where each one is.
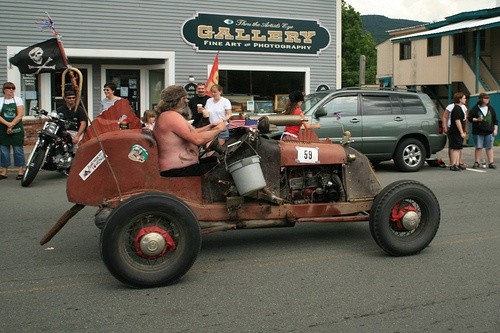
[259,87,447,172]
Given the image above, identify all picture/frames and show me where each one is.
[274,94,290,113]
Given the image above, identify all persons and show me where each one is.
[282,91,320,140]
[141,82,232,177]
[99,82,121,113]
[443,92,469,171]
[41,91,88,174]
[467,93,498,170]
[0,82,26,180]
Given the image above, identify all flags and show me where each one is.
[9,38,68,76]
[205,53,219,97]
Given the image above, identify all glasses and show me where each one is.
[4,86,15,90]
[103,88,110,92]
[66,96,77,100]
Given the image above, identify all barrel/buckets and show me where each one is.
[224,140,267,197]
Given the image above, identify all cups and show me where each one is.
[196,104,203,113]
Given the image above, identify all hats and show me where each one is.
[160,84,187,102]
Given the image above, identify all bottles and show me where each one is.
[482,159,486,169]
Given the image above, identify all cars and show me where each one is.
[40,98,441,288]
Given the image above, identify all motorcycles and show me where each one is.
[21,106,76,186]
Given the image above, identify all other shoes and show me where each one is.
[0,172,9,180]
[472,161,481,169]
[16,172,25,180]
[449,164,466,171]
[488,162,498,169]
